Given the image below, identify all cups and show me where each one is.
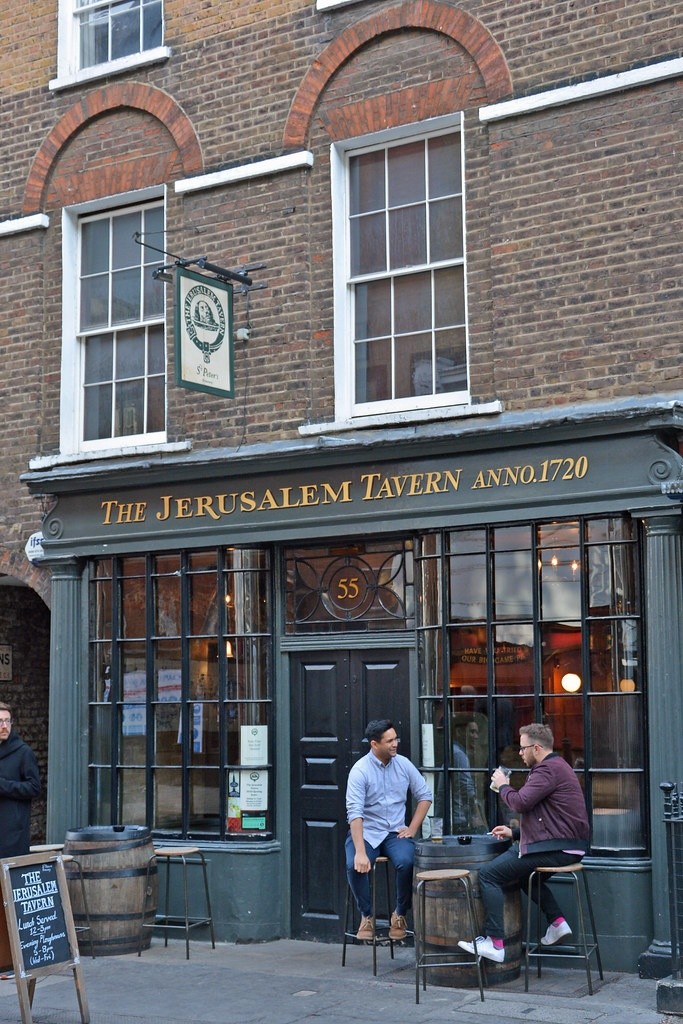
[490,766,512,793]
[430,817,443,845]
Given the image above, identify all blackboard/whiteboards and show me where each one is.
[0,851,81,980]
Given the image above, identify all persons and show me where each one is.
[345,720,433,940]
[437,722,487,836]
[457,723,591,963]
[0,702,42,980]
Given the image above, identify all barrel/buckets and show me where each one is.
[64,824,159,956]
[412,834,524,989]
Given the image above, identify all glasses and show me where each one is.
[520,744,542,750]
[0,719,13,726]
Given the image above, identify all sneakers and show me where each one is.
[389,911,408,939]
[357,915,373,940]
[540,921,572,946]
[458,936,505,963]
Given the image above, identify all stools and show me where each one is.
[59,854,95,959]
[30,843,65,854]
[342,857,416,977]
[416,869,489,1004]
[522,863,603,996]
[138,846,215,959]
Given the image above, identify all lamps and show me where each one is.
[216,640,234,658]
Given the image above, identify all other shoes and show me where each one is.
[0,970,15,979]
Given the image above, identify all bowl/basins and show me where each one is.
[113,825,126,832]
[458,835,471,844]
